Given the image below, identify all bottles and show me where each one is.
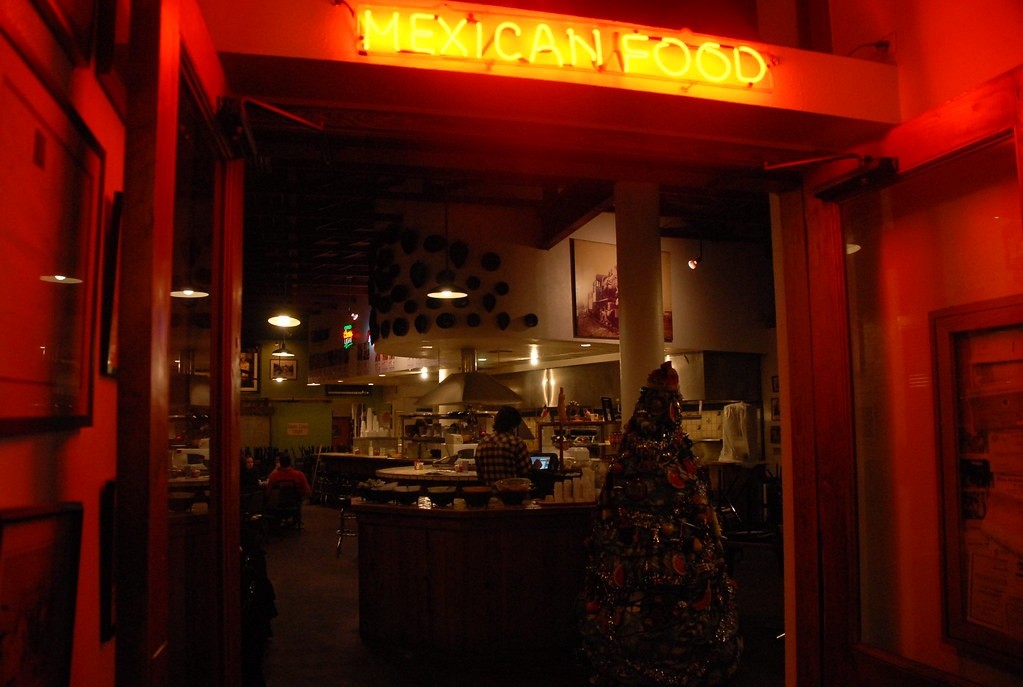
[175,450,184,471]
[609,432,624,448]
[369,441,373,455]
[355,447,360,455]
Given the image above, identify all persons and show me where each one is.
[240,453,311,529]
[474,406,543,486]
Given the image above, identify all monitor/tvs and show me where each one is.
[530,452,556,472]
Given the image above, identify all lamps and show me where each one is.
[425,181,469,300]
[272,337,295,357]
[268,298,301,329]
[169,118,209,298]
[688,232,704,270]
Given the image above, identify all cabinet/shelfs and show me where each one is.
[399,411,493,459]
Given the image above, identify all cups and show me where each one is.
[463,435,473,444]
[546,495,553,502]
[462,461,469,473]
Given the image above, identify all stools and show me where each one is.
[335,498,360,558]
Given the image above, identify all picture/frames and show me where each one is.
[771,398,780,421]
[270,359,298,379]
[770,426,781,443]
[772,375,779,392]
[240,343,262,393]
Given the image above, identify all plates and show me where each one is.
[463,487,491,492]
[376,482,398,491]
[428,487,456,493]
[394,485,421,492]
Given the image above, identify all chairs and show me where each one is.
[264,481,302,534]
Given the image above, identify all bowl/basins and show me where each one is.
[496,478,530,491]
